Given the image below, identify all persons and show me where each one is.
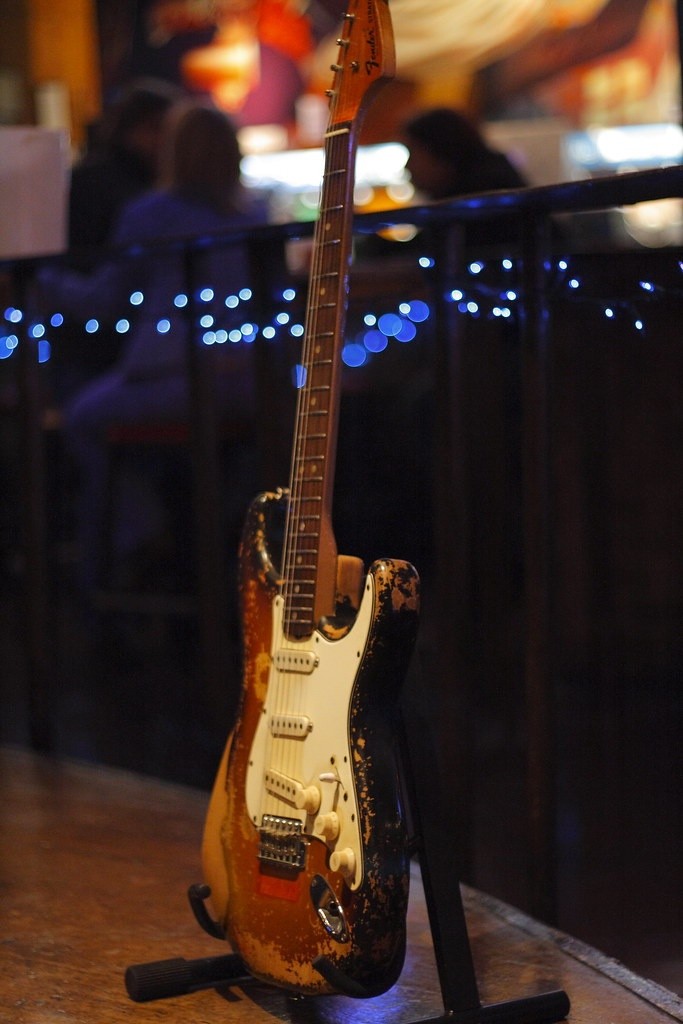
[64,88,526,254]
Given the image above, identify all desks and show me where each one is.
[0,747,683,1024]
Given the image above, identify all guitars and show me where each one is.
[196,0,425,1000]
